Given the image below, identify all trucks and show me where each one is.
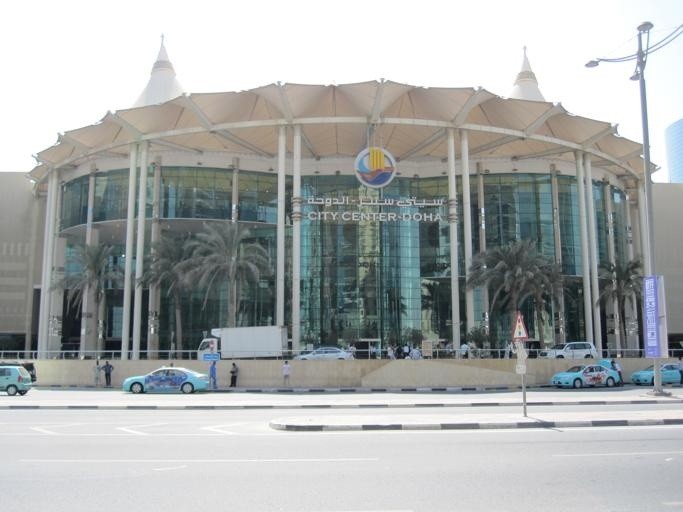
[196,325,291,362]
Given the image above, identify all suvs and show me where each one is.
[536,341,599,360]
[0,361,38,386]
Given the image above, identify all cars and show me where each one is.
[122,367,211,394]
[0,366,32,395]
[631,361,683,385]
[550,360,622,389]
[292,346,355,360]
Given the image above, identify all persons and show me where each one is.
[408,346,421,359]
[230,362,239,387]
[678,355,683,384]
[282,361,290,386]
[210,361,217,385]
[460,341,469,359]
[94,360,114,388]
[389,343,396,360]
[350,343,356,351]
[611,360,624,387]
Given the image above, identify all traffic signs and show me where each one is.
[203,353,220,362]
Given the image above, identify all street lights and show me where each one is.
[583,20,683,399]
